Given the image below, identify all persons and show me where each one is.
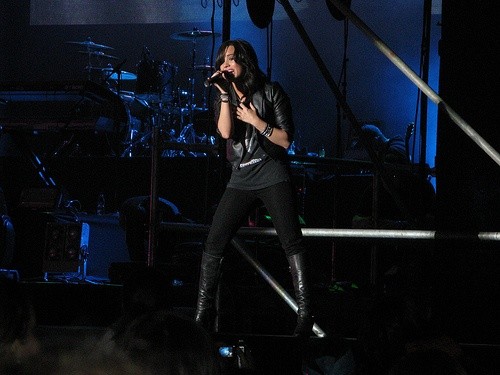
[361,124,406,164]
[192,39,309,338]
[0,278,472,375]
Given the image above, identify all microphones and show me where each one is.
[204,69,229,86]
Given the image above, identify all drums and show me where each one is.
[76,84,154,156]
[140,91,194,119]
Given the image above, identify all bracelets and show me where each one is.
[220,93,230,102]
[260,123,273,137]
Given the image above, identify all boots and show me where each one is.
[286,250,314,337]
[189,251,224,332]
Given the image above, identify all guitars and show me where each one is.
[405,121,414,159]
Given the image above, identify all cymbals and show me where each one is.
[169,31,221,40]
[97,69,137,81]
[79,51,120,60]
[189,64,216,71]
[64,40,114,51]
[81,66,113,72]
